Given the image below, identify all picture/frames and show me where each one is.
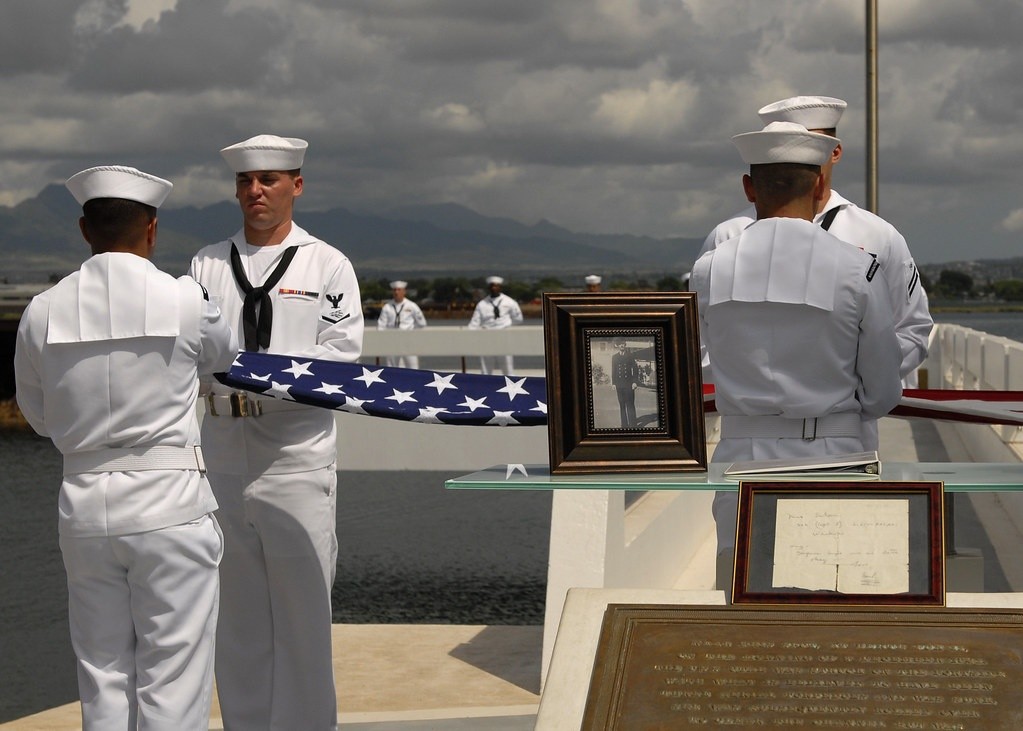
[731,480,948,610]
[540,290,712,475]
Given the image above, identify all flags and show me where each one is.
[215,349,1023,428]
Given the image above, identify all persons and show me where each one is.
[16,164,226,731]
[468,276,524,376]
[645,364,650,376]
[378,281,427,370]
[638,364,644,381]
[691,121,903,608]
[681,272,691,292]
[612,337,639,430]
[583,275,602,292]
[189,134,365,731]
[699,95,933,380]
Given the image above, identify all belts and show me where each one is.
[60,444,209,475]
[720,414,863,439]
[200,391,317,418]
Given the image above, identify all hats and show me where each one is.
[64,165,173,209]
[757,96,848,129]
[731,121,840,167]
[584,275,601,285]
[614,338,626,344]
[486,276,504,285]
[219,134,309,172]
[681,272,691,283]
[389,281,408,289]
